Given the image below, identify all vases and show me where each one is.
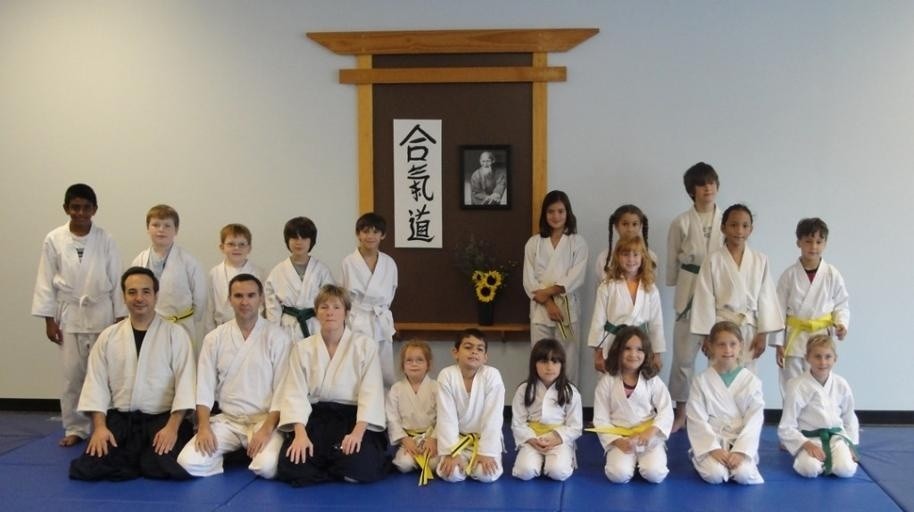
[477,299,494,326]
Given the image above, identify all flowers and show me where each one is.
[454,236,512,304]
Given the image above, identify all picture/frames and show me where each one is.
[458,144,512,210]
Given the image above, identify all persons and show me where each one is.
[174,275,288,481]
[685,321,765,486]
[590,234,665,380]
[595,205,657,287]
[385,338,440,473]
[434,328,504,484]
[263,217,336,342]
[523,190,587,389]
[341,213,398,411]
[777,336,859,478]
[510,338,583,481]
[469,153,505,203]
[689,205,784,376]
[131,206,207,423]
[769,218,849,452]
[68,266,198,480]
[275,284,396,485]
[203,223,269,340]
[33,183,125,447]
[591,326,674,484]
[663,162,724,433]
